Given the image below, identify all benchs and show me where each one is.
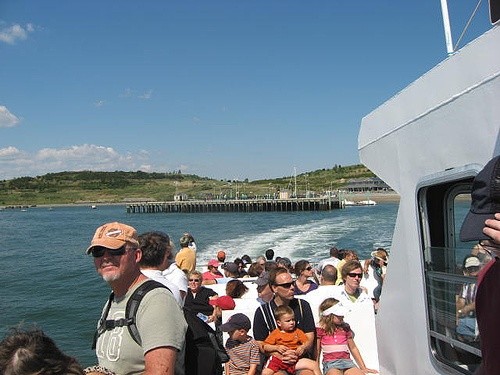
[202,260,380,374]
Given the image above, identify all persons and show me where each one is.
[0,329,116,375]
[454,240,494,336]
[253,267,316,375]
[137,231,183,305]
[161,241,188,301]
[184,270,217,323]
[227,280,244,298]
[85,222,188,375]
[252,271,275,305]
[219,313,261,375]
[175,233,197,277]
[209,296,236,329]
[260,305,322,375]
[459,155,500,375]
[289,247,390,307]
[201,249,291,285]
[314,298,378,375]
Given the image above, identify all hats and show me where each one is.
[322,302,351,317]
[460,155,500,240]
[464,257,481,268]
[252,271,271,286]
[86,222,138,253]
[371,250,389,263]
[218,313,251,332]
[221,262,238,273]
[207,259,221,267]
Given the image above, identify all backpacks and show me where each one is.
[92,279,230,375]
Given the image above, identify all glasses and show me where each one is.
[304,267,311,271]
[479,240,500,257]
[189,279,199,282]
[347,272,362,278]
[275,281,296,289]
[91,247,136,257]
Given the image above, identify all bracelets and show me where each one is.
[458,310,461,314]
